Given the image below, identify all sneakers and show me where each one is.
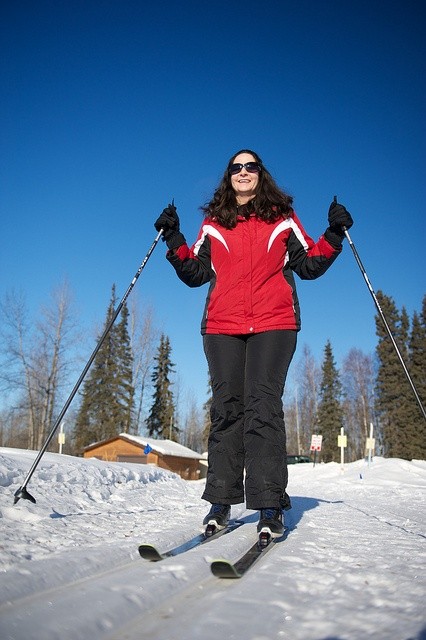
[203,504,230,531]
[257,509,284,539]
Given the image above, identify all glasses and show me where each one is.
[229,162,262,174]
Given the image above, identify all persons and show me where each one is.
[153,146,355,542]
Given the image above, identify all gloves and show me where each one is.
[324,201,354,247]
[154,203,186,251]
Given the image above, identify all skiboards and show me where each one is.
[137,519,289,579]
